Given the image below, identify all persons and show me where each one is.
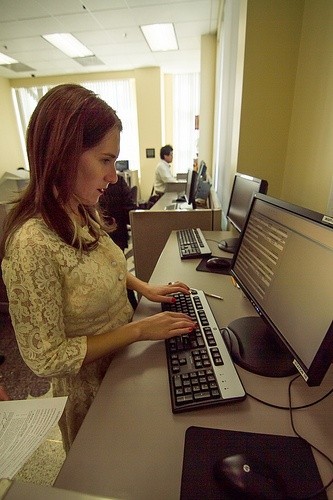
[98,175,137,255]
[153,145,178,195]
[2,84,195,457]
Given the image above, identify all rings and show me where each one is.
[168,282,174,286]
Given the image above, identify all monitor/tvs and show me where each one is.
[229,192,333,386]
[217,172,268,253]
[115,160,129,170]
[184,162,207,205]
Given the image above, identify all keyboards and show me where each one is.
[161,289,247,414]
[176,227,211,259]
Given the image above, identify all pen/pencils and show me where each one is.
[168,282,223,299]
[232,277,240,290]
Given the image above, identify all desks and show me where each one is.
[130,182,222,302]
[53,229,333,500]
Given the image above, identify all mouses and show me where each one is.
[176,198,186,202]
[213,454,286,500]
[205,257,230,268]
